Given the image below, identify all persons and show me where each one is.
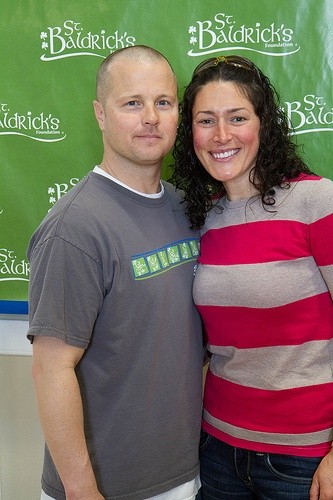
[172,55,331,499]
[25,46,209,500]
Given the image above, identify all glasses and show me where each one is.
[193,55,262,85]
